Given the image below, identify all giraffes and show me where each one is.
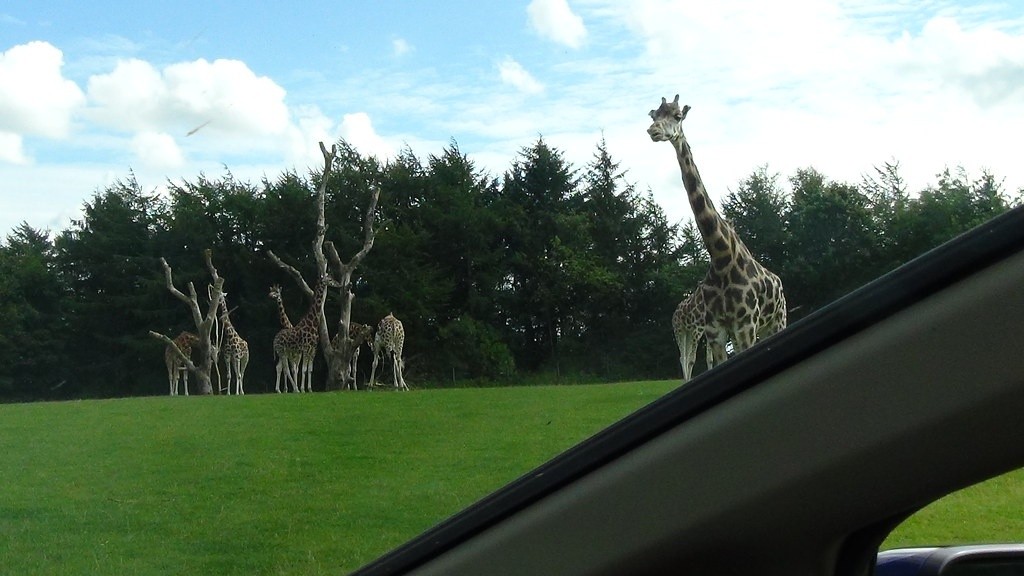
[164,331,218,396]
[268,274,340,393]
[332,322,375,391]
[219,297,249,395]
[645,93,788,383]
[366,312,410,393]
